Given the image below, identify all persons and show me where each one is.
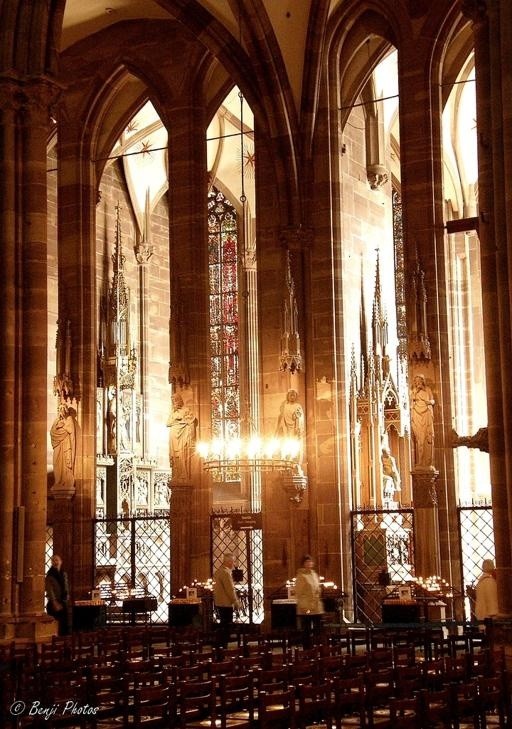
[296,554,324,636]
[44,554,69,637]
[166,392,196,482]
[381,447,401,497]
[410,373,436,472]
[50,403,76,488]
[274,388,305,439]
[214,553,242,642]
[475,559,499,645]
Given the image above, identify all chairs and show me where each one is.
[1,627,512,726]
